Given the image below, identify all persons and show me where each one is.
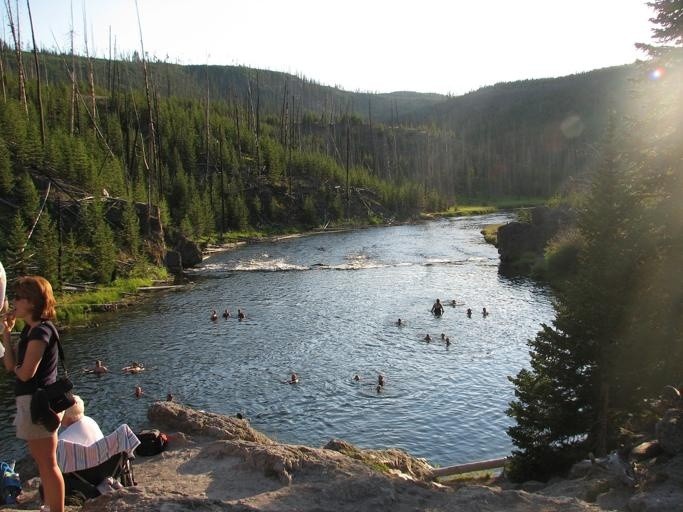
[235,412,243,420]
[167,393,174,401]
[122,361,142,373]
[0,275,66,512]
[60,395,104,448]
[355,374,384,392]
[94,359,107,372]
[212,309,246,321]
[398,298,488,345]
[134,386,144,395]
[290,374,298,384]
[0,262,8,360]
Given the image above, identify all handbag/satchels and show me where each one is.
[31,321,76,413]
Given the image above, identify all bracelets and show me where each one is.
[13,364,20,374]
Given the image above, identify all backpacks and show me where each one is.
[135,428,169,456]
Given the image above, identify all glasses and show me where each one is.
[14,294,26,301]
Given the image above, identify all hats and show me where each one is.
[28,389,60,433]
